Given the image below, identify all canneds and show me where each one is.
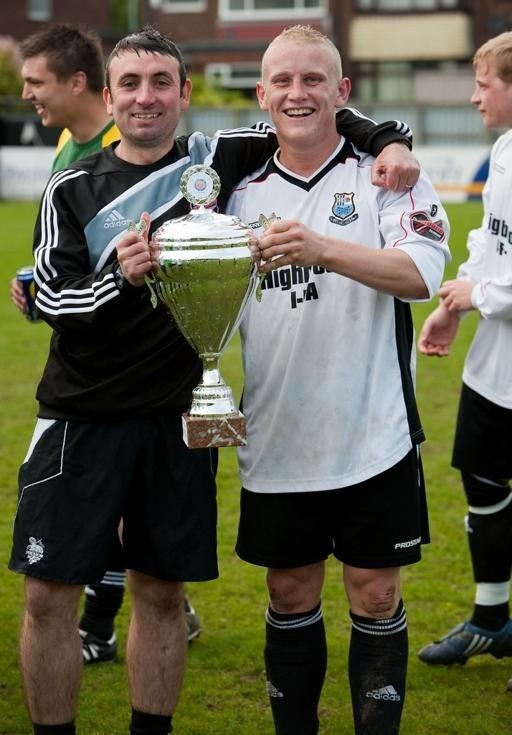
[17,267,45,323]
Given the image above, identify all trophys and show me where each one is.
[128,164,279,449]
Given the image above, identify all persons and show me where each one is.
[417,29,512,666]
[224,23,452,735]
[10,22,202,667]
[9,26,420,735]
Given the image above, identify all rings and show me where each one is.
[405,183,412,187]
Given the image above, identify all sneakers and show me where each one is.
[418,619,512,666]
[183,606,202,644]
[78,625,118,665]
